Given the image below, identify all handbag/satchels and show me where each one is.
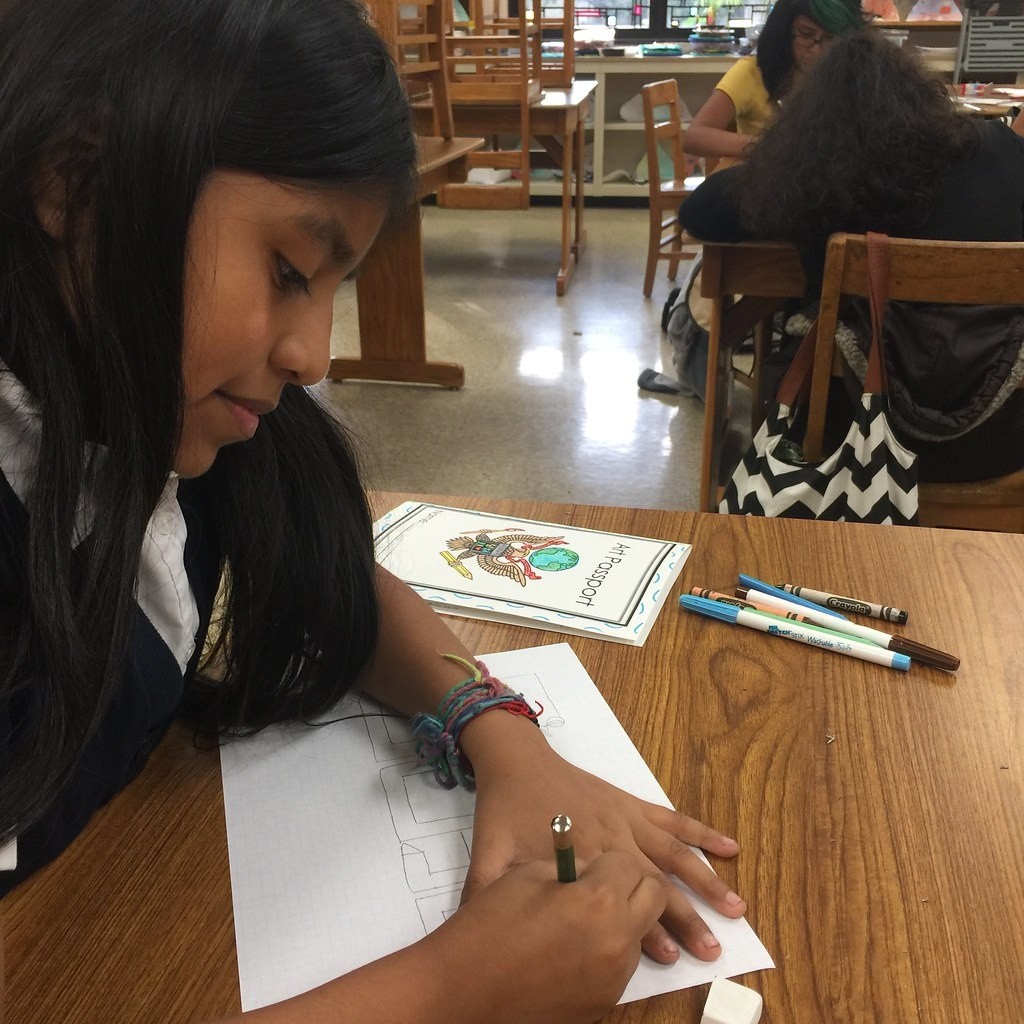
[713,231,921,527]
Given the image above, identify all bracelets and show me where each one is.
[409,650,544,793]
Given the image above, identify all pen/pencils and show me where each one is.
[550,815,577,882]
[689,586,822,626]
[734,586,961,671]
[745,607,885,650]
[775,582,909,625]
[737,574,849,620]
[680,593,912,671]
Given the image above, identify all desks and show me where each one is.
[1,491,1024,1024]
[409,78,598,296]
[943,84,1024,124]
[325,137,485,393]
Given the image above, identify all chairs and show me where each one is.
[951,9,1024,83]
[639,78,706,298]
[804,232,1024,534]
[357,0,574,209]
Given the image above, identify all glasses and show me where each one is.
[791,30,835,51]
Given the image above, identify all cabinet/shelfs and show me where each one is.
[431,54,748,198]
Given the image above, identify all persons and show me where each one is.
[2,0,749,1023]
[678,1,1023,482]
